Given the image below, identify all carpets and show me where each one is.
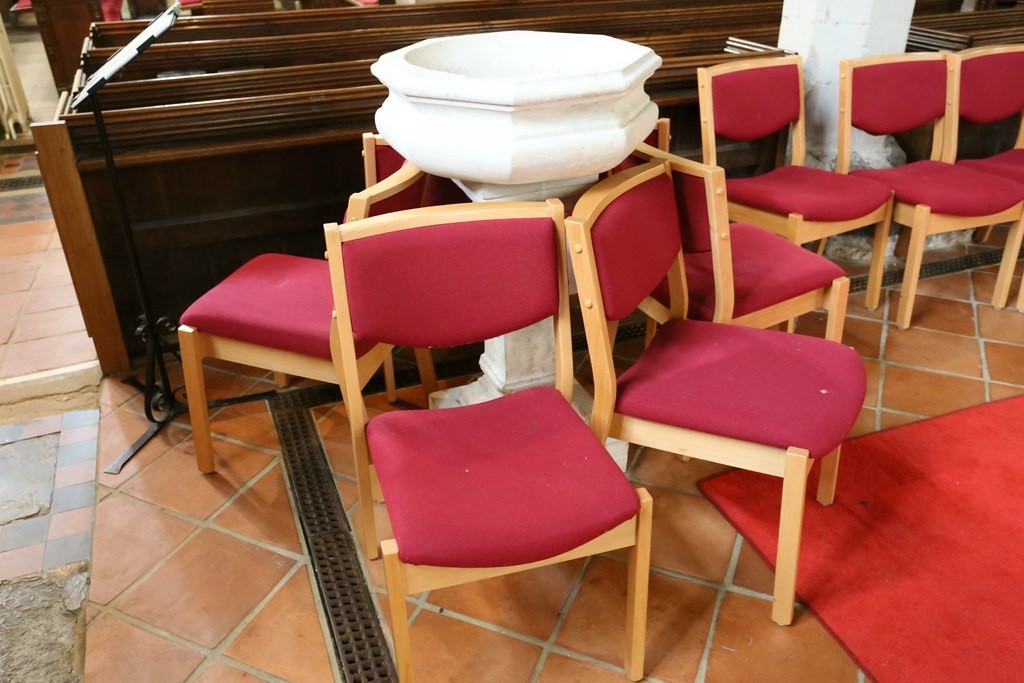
[698,394,1024,683]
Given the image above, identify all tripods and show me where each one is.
[70,7,265,475]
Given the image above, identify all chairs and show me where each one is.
[175,43,1024,683]
